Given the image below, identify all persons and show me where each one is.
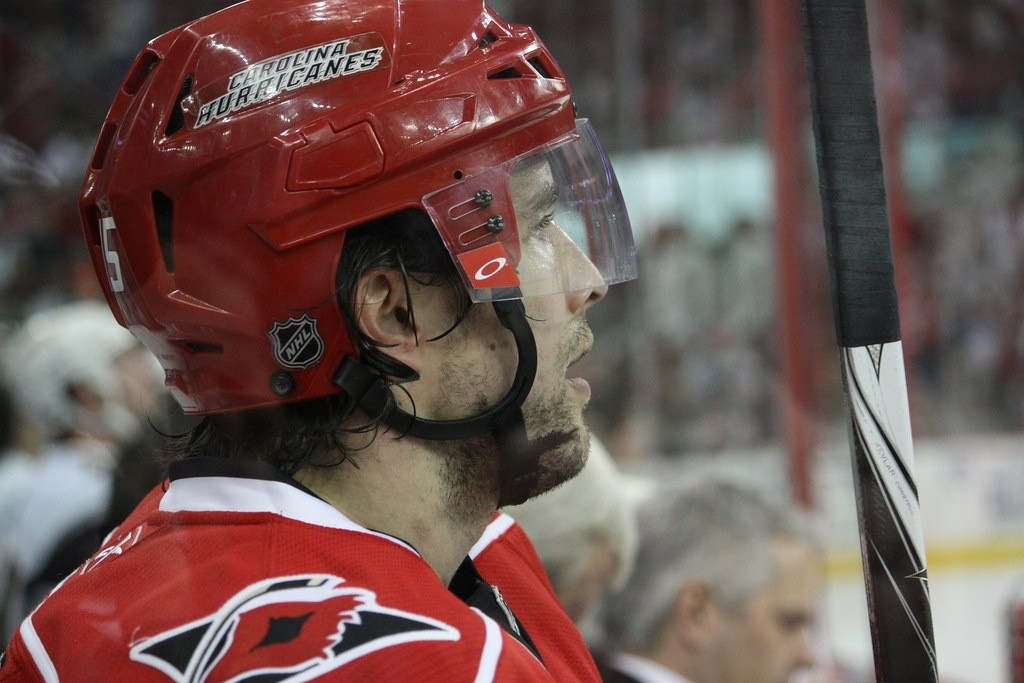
[602,478,819,683]
[0,298,171,627]
[1,0,607,682]
[500,437,636,619]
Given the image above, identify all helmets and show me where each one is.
[1,296,144,435]
[79,0,573,416]
